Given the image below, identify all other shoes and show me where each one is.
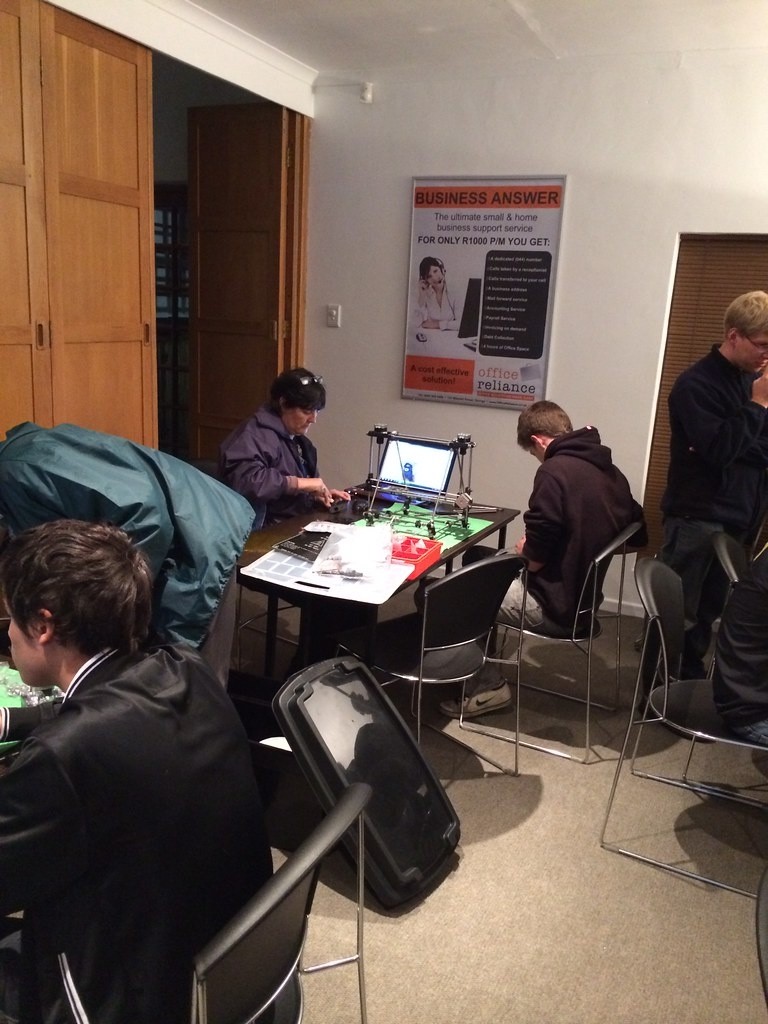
[639,685,656,718]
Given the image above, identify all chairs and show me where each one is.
[190,782,375,1024]
[336,554,533,775]
[459,519,647,764]
[682,531,768,807]
[599,557,768,900]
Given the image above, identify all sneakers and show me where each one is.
[438,678,513,719]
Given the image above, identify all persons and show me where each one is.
[0,419,256,651]
[638,290,768,719]
[439,400,649,718]
[216,367,351,529]
[711,548,768,746]
[0,518,274,1024]
[416,257,463,331]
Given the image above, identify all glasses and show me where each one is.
[297,374,324,385]
[745,332,768,353]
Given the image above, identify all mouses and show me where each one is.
[417,333,427,342]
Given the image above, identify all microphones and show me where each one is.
[439,270,446,283]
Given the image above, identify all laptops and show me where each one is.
[344,433,458,507]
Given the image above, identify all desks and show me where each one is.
[226,493,520,727]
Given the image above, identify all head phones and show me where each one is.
[420,258,446,286]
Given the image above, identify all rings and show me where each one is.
[321,486,327,493]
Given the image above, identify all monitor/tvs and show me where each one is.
[457,279,481,351]
